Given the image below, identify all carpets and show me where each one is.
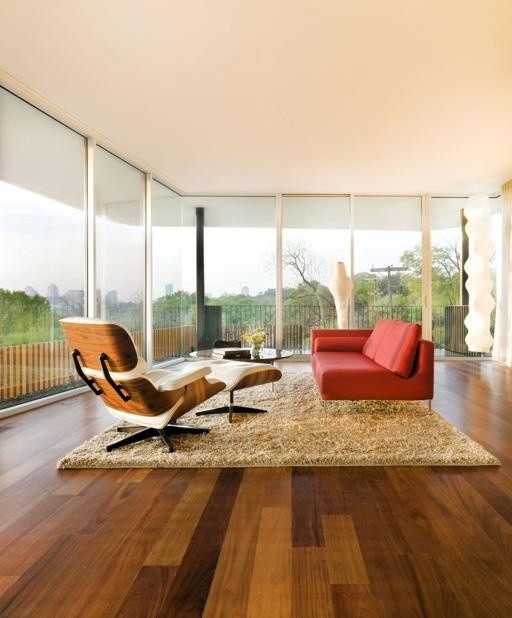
[56,373,501,470]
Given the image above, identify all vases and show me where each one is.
[251,344,260,362]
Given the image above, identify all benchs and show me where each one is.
[199,359,280,423]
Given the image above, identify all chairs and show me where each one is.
[213,340,240,348]
[58,318,224,451]
[223,350,251,361]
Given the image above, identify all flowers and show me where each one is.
[240,329,266,358]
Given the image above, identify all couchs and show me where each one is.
[311,329,435,413]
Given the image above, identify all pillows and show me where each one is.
[312,337,368,352]
